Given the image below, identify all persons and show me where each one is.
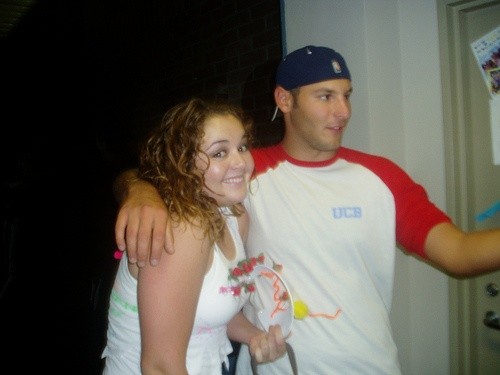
[115,45,500,375]
[99,97,287,375]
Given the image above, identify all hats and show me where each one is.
[276,45,352,90]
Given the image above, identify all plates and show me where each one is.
[244,265,294,340]
